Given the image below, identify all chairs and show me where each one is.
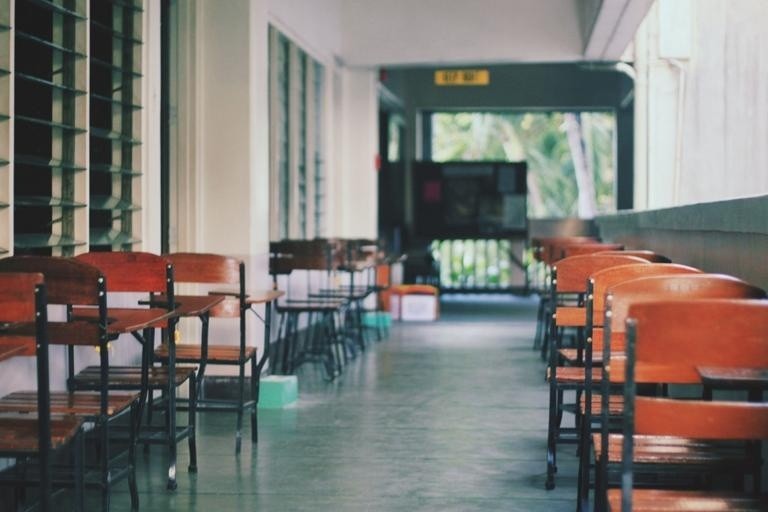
[541,243,625,361]
[605,298,768,512]
[0,271,88,512]
[555,249,663,430]
[590,272,768,511]
[531,235,602,351]
[146,251,258,455]
[575,261,715,512]
[268,237,391,383]
[66,249,197,490]
[0,249,142,510]
[542,253,654,492]
